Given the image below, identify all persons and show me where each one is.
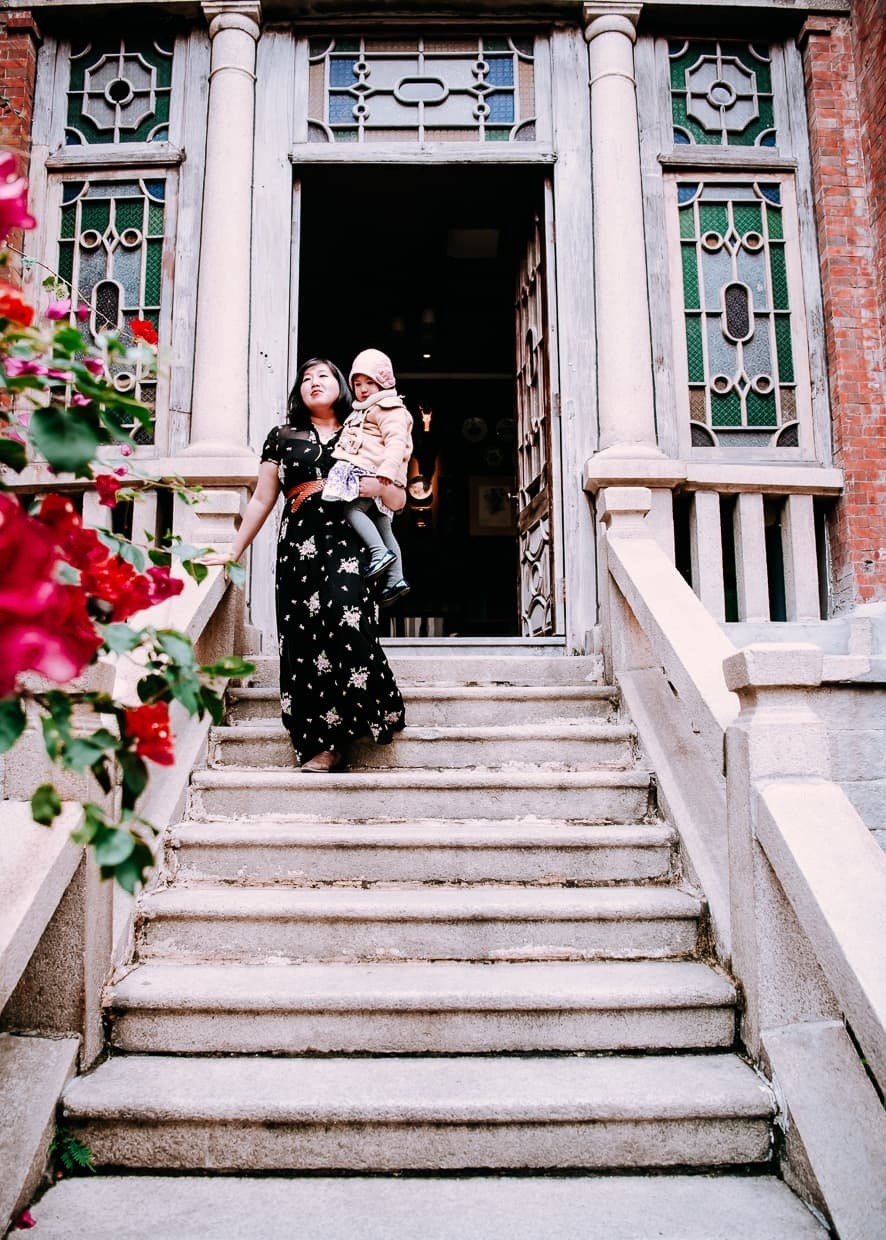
[201,355,406,774]
[321,348,414,603]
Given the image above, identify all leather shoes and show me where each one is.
[379,579,411,608]
[362,550,397,580]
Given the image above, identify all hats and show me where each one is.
[349,349,396,389]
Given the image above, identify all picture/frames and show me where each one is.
[469,476,516,536]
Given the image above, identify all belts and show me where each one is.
[286,477,328,514]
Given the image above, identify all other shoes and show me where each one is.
[301,751,347,774]
